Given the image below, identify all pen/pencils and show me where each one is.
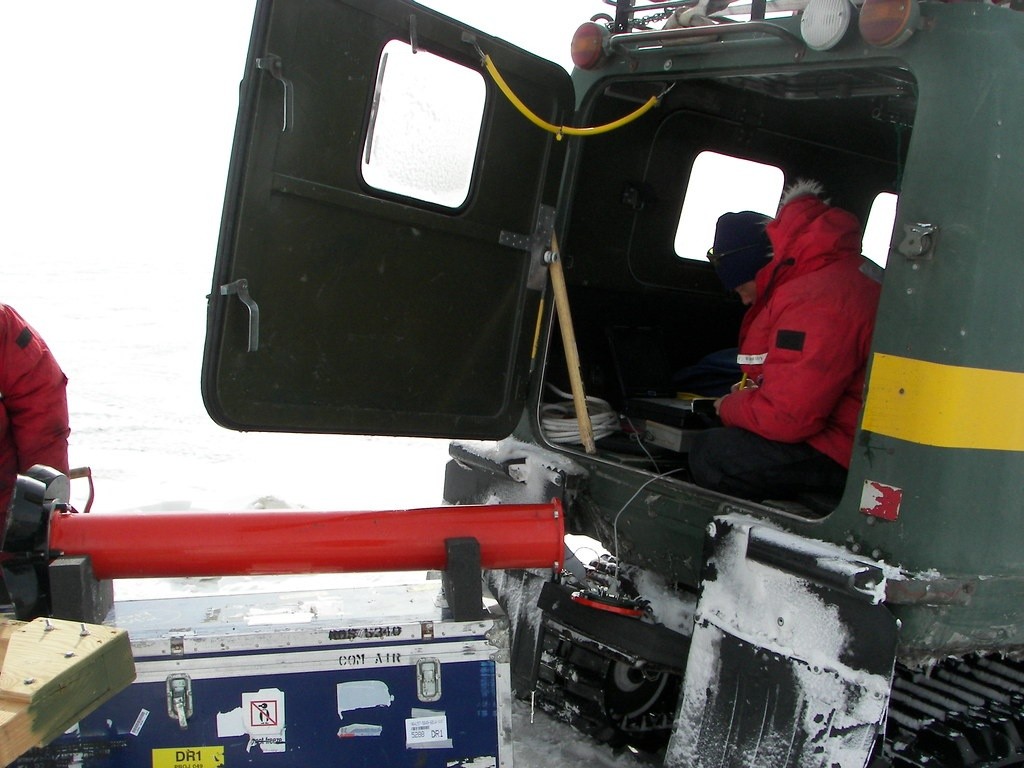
[739,372,747,391]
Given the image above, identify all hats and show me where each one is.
[712,212,776,293]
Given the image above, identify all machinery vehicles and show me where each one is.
[195,0,1024,768]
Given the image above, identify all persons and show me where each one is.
[0,303,71,603]
[686,179,884,518]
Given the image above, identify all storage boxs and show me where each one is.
[606,323,729,453]
[53,577,503,768]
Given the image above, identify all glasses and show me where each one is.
[707,246,750,267]
